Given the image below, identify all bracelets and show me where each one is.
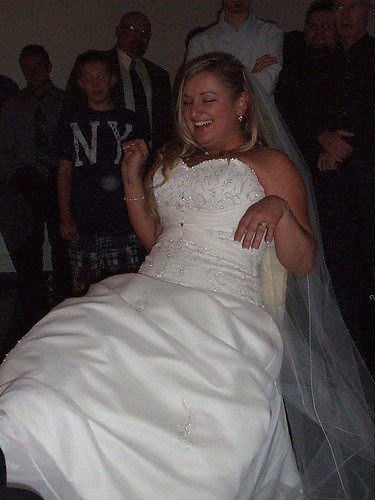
[124,194,145,200]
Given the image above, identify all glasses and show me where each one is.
[120,24,153,39]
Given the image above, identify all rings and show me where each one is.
[260,222,268,229]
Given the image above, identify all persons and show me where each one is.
[0,0,374,373]
[0,52,375,500]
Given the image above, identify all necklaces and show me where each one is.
[199,150,234,156]
